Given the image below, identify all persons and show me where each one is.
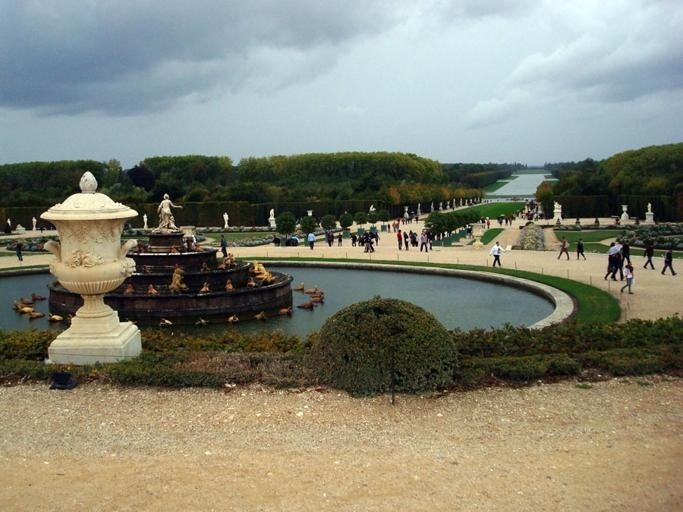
[169,263,186,292]
[15,239,22,261]
[576,237,587,261]
[155,193,183,226]
[490,240,506,267]
[480,200,538,230]
[220,234,227,257]
[4,223,10,233]
[663,248,677,276]
[643,244,657,270]
[603,238,636,294]
[307,214,439,253]
[556,236,569,260]
